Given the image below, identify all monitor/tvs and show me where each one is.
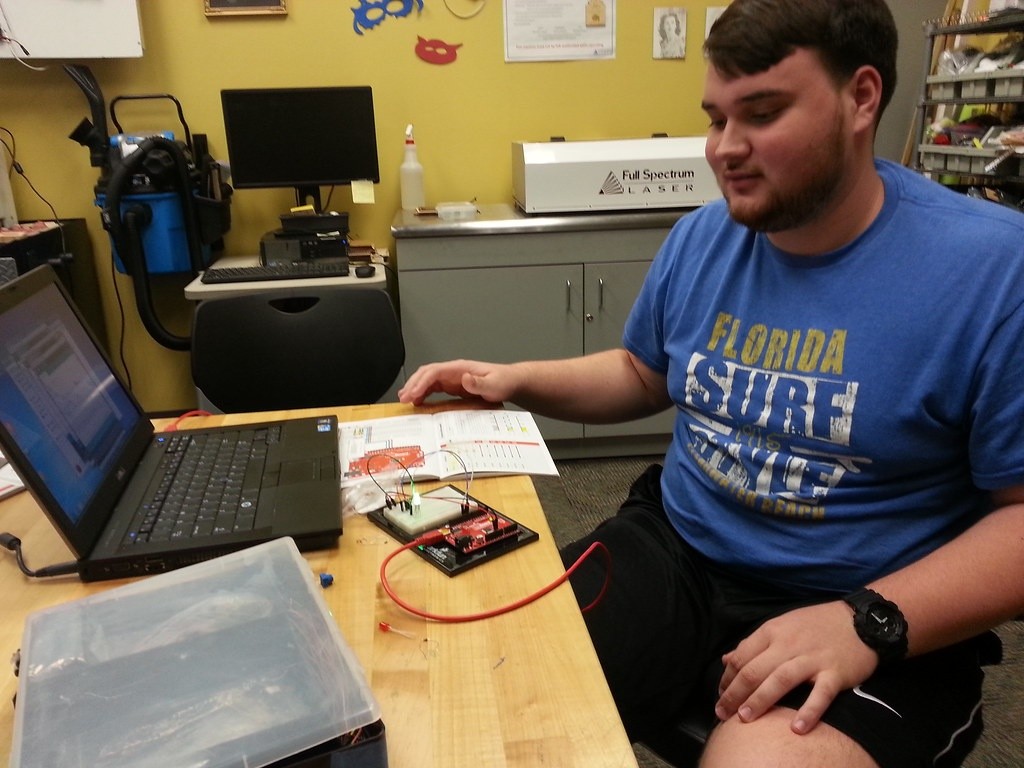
[222,85,381,215]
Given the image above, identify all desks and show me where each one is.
[0,396,640,768]
[184,253,386,300]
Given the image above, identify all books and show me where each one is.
[337,411,561,486]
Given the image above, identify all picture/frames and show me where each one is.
[203,0,286,17]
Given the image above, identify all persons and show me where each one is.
[398,1,1024,768]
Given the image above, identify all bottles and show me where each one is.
[108,131,175,147]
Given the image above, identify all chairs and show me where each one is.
[191,287,406,414]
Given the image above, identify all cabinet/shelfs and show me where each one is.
[1,219,113,360]
[390,204,695,460]
[913,13,1024,182]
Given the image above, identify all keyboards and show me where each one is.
[200,258,349,285]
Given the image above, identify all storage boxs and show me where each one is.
[9,536,388,767]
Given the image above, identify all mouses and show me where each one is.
[355,266,376,279]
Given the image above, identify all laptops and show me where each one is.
[0,261,342,584]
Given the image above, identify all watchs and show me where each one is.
[844,587,909,679]
